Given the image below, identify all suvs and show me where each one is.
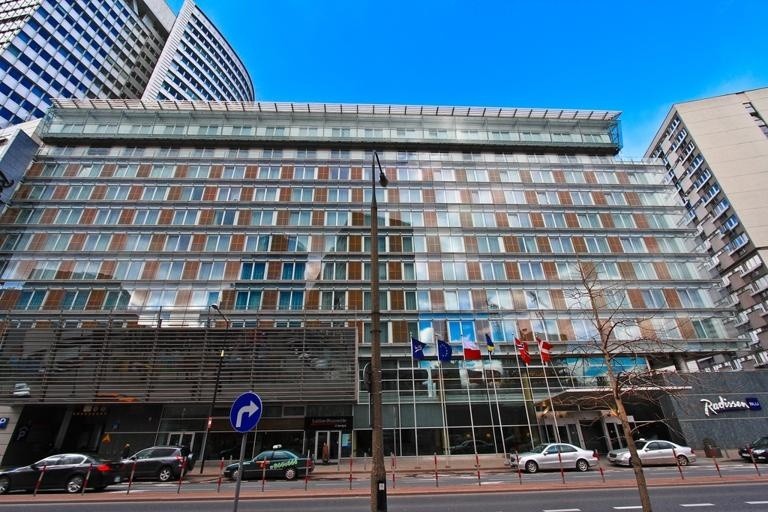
[106,446,193,482]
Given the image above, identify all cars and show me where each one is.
[222,449,315,482]
[510,438,568,453]
[738,436,767,463]
[507,443,598,474]
[0,452,113,494]
[443,440,492,454]
[216,442,257,459]
[606,438,695,469]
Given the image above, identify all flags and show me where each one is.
[464,340,481,361]
[439,339,453,366]
[515,338,532,365]
[536,336,552,362]
[485,334,496,352]
[413,339,426,360]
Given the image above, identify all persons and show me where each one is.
[122,443,130,458]
[321,441,329,465]
[181,443,190,457]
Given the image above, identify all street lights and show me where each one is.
[368,148,391,510]
[198,304,229,474]
